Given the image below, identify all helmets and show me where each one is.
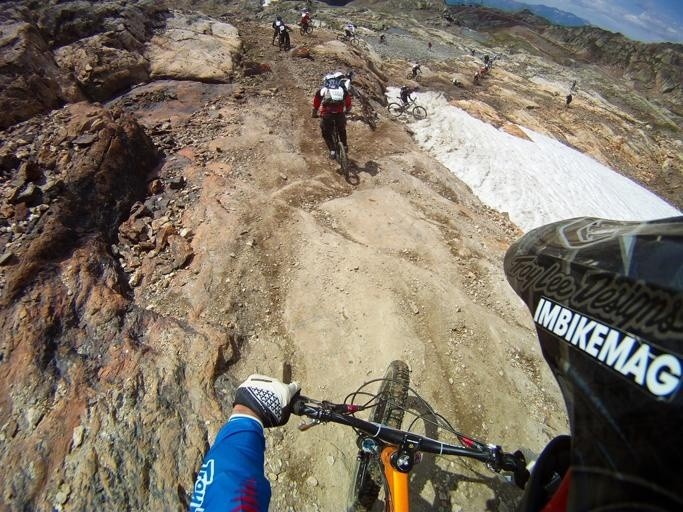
[502,214,682,481]
[323,71,344,81]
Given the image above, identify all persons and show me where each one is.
[270,15,293,47]
[301,11,313,32]
[311,72,352,161]
[571,79,576,90]
[186,215,683,511]
[399,84,419,112]
[345,72,360,99]
[411,64,422,79]
[427,40,433,52]
[471,48,497,81]
[565,94,573,107]
[449,78,465,88]
[380,33,385,42]
[344,23,358,42]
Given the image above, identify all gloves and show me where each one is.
[230,371,301,429]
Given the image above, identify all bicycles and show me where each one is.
[336,30,359,47]
[289,360,530,512]
[300,21,313,36]
[311,111,353,182]
[388,97,427,120]
[407,72,422,82]
[355,87,376,130]
